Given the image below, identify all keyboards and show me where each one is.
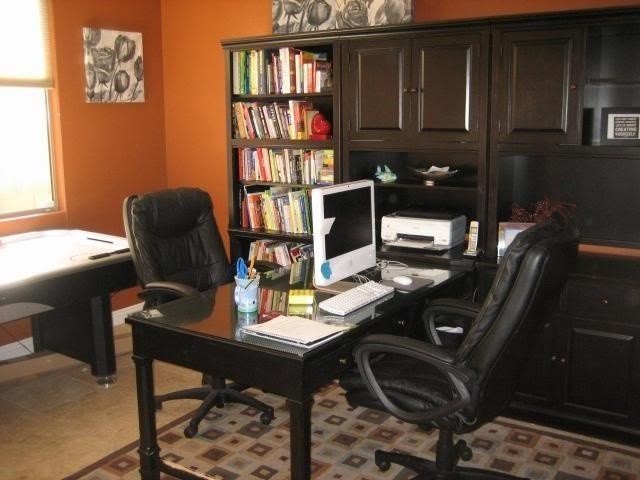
[318,281,394,317]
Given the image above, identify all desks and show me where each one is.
[0,227,138,386]
[124,252,470,480]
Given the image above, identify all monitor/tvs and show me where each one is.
[310,180,376,294]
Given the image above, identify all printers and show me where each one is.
[380,211,466,250]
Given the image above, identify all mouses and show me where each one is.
[394,275,413,285]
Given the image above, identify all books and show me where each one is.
[231,45,334,268]
[239,314,347,348]
[257,254,320,324]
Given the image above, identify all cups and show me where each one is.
[234,312,259,343]
[233,274,261,313]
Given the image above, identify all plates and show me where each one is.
[406,165,461,185]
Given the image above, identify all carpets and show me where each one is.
[62,379,639,480]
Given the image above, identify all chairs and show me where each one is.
[122,188,283,439]
[339,218,581,479]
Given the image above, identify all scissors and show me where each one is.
[236,257,247,279]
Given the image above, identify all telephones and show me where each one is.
[462,221,480,256]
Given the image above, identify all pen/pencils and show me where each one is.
[87,238,113,243]
[248,256,259,279]
